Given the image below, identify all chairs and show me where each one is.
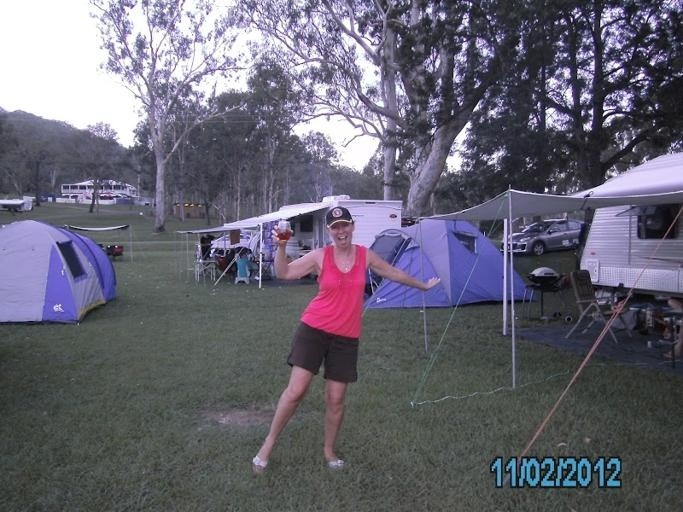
[194,242,216,282]
[564,270,632,343]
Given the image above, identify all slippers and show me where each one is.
[252,455,268,470]
[328,460,345,470]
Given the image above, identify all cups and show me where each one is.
[277,220,291,242]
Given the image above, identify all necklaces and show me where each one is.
[336,246,354,272]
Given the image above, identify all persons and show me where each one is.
[246,206,440,471]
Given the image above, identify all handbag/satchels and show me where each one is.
[237,254,251,278]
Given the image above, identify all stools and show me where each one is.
[234,277,249,284]
[521,285,567,321]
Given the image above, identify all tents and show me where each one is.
[361,219,535,313]
[0,219,116,331]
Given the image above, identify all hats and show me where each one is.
[326,207,353,228]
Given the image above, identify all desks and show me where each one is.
[254,258,273,280]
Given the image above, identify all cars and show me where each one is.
[55,191,137,201]
[501,219,585,255]
[401,216,416,226]
[195,230,257,260]
